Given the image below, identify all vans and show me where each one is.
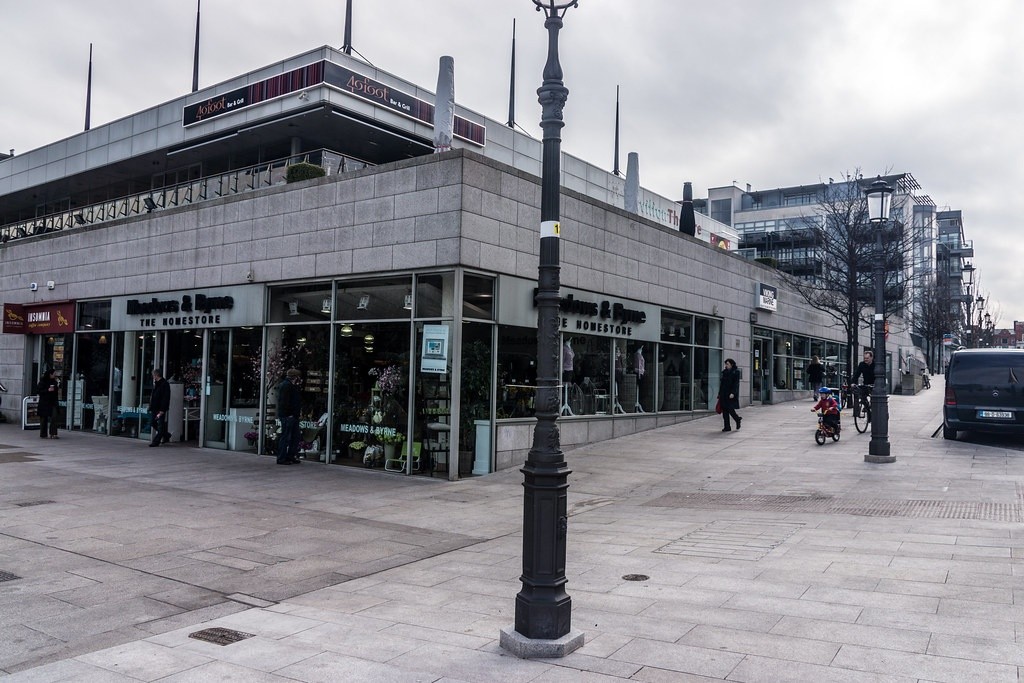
[943,345,1024,440]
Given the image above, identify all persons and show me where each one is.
[276,369,301,465]
[148,368,172,447]
[850,351,875,412]
[615,349,623,384]
[562,341,575,385]
[633,349,645,385]
[718,359,742,432]
[806,355,825,401]
[36,367,59,440]
[811,387,842,438]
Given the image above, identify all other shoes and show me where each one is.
[722,427,731,432]
[862,402,869,411]
[42,435,60,439]
[835,425,840,434]
[277,456,301,465]
[162,433,171,444]
[148,443,159,447]
[736,417,742,429]
[813,396,817,401]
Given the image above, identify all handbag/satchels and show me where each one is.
[716,400,722,414]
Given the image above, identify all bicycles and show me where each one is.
[811,409,840,446]
[921,366,932,389]
[853,384,874,434]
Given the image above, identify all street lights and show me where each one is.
[984,312,991,348]
[863,174,897,462]
[960,261,977,349]
[976,295,986,348]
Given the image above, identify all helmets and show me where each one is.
[817,386,831,393]
